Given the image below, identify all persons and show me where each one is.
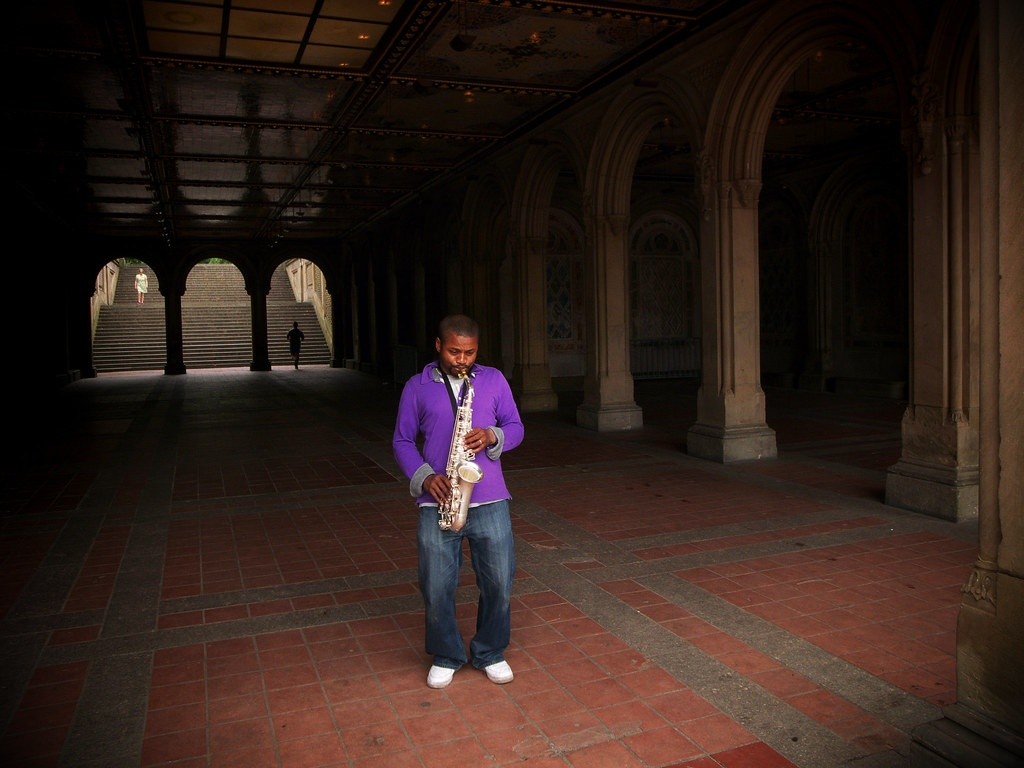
[287,322,304,369]
[392,316,524,689]
[135,267,148,304]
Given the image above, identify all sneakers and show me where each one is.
[427,665,462,688]
[485,660,515,684]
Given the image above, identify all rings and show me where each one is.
[478,440,483,445]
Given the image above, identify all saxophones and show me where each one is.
[437,367,485,532]
